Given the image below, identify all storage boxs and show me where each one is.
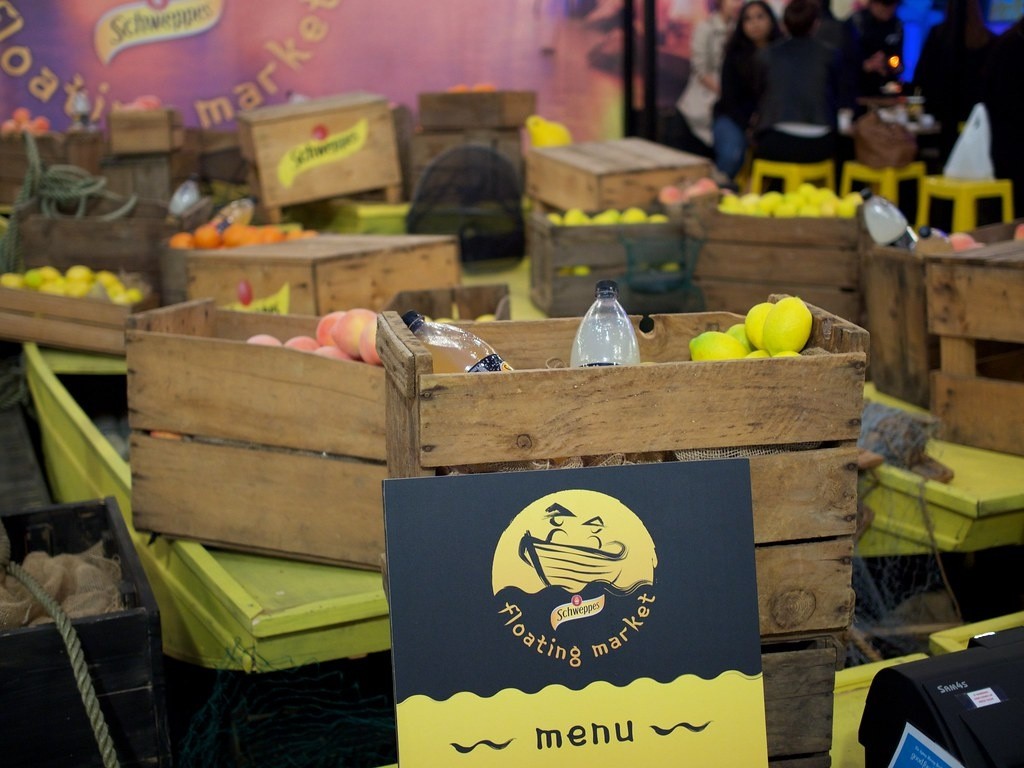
[522,136,713,213]
[417,81,537,128]
[869,220,1024,464]
[526,208,693,322]
[120,296,389,585]
[404,130,525,192]
[233,91,407,229]
[376,288,867,762]
[183,233,457,320]
[2,264,161,358]
[682,188,877,329]
[99,156,172,210]
[106,111,177,156]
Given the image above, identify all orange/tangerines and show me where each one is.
[168,222,317,248]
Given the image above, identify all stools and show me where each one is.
[751,159,834,198]
[839,162,926,217]
[915,176,1012,234]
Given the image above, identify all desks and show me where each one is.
[24,334,1024,671]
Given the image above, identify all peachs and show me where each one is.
[244,309,382,368]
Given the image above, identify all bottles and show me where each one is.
[72,86,92,133]
[164,172,199,224]
[286,89,308,103]
[208,192,261,233]
[401,309,516,375]
[568,278,641,370]
[918,224,949,241]
[860,190,922,253]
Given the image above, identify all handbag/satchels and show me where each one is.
[856,111,917,168]
[944,101,998,181]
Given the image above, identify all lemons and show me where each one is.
[720,184,862,221]
[542,204,666,225]
[689,296,814,359]
[0,265,143,304]
